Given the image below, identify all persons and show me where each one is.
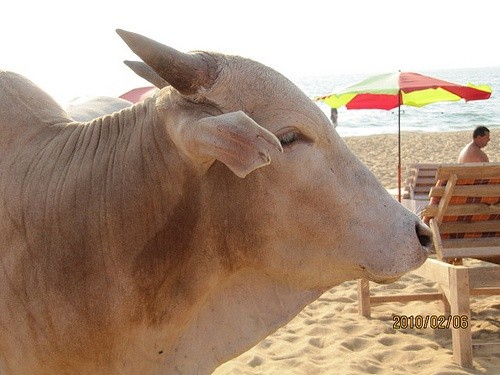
[458,125,490,164]
[329,107,337,129]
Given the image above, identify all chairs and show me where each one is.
[359,164,500,369]
[404,162,441,199]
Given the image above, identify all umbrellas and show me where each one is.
[313,65,492,205]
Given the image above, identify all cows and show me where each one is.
[0,28,434,375]
